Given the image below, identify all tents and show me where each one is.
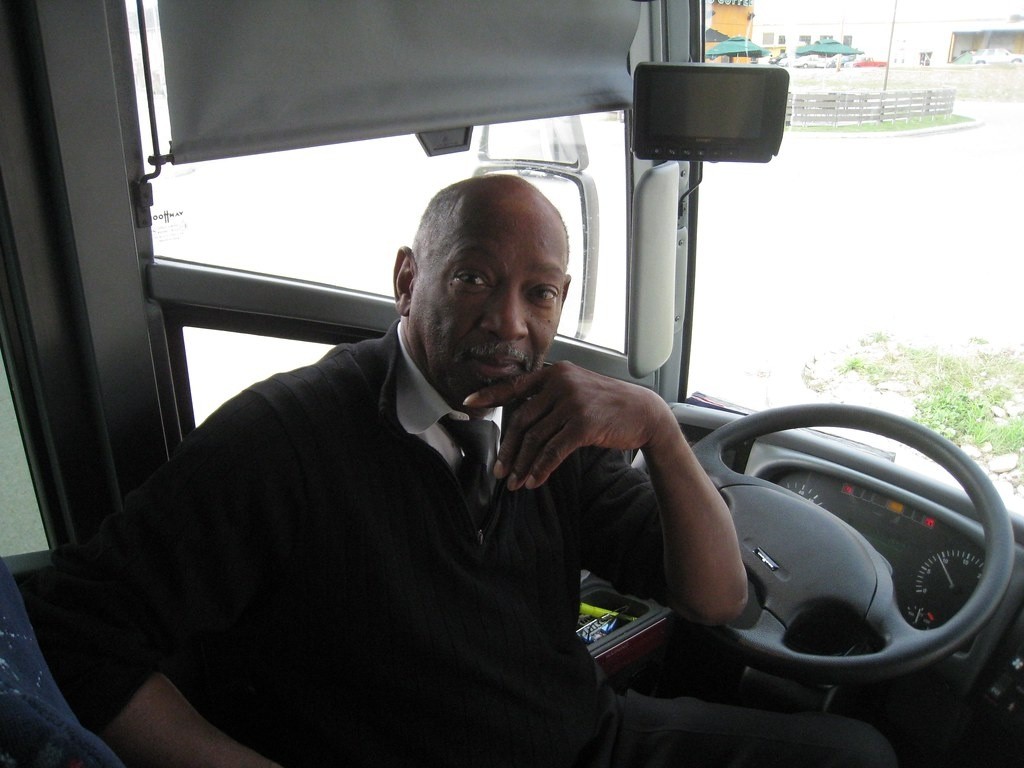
[796,39,864,72]
[705,37,770,65]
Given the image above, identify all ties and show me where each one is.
[438,414,498,529]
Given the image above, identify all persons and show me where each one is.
[18,176,899,768]
